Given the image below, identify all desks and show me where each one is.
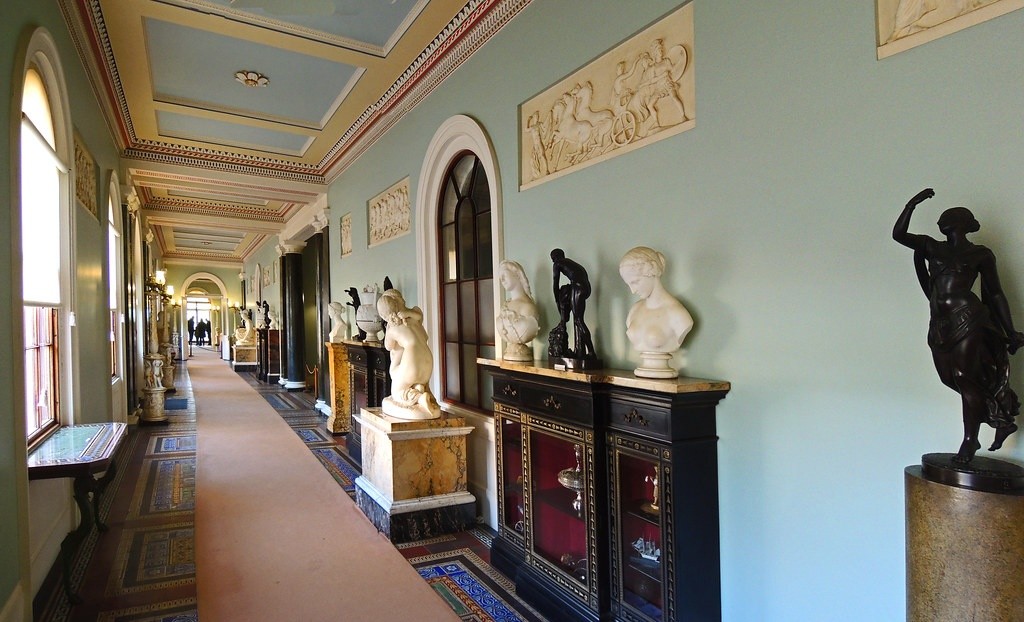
[26,422,130,551]
[164,399,187,409]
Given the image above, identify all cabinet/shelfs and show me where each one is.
[338,339,392,465]
[475,358,731,622]
[252,327,268,381]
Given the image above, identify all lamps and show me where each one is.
[227,301,240,310]
[145,271,182,308]
[212,306,220,312]
[236,71,269,88]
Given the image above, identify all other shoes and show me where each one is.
[208,344,210,345]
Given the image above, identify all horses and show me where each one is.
[544,81,616,174]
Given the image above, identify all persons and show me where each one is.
[496,260,539,344]
[377,289,435,416]
[328,302,347,336]
[344,287,368,339]
[268,311,277,328]
[371,186,408,240]
[238,306,253,341]
[158,311,170,327]
[263,301,271,325]
[526,109,554,181]
[144,360,166,388]
[194,319,211,346]
[613,39,688,130]
[551,249,598,358]
[188,316,194,345]
[618,246,694,353]
[893,188,1024,462]
